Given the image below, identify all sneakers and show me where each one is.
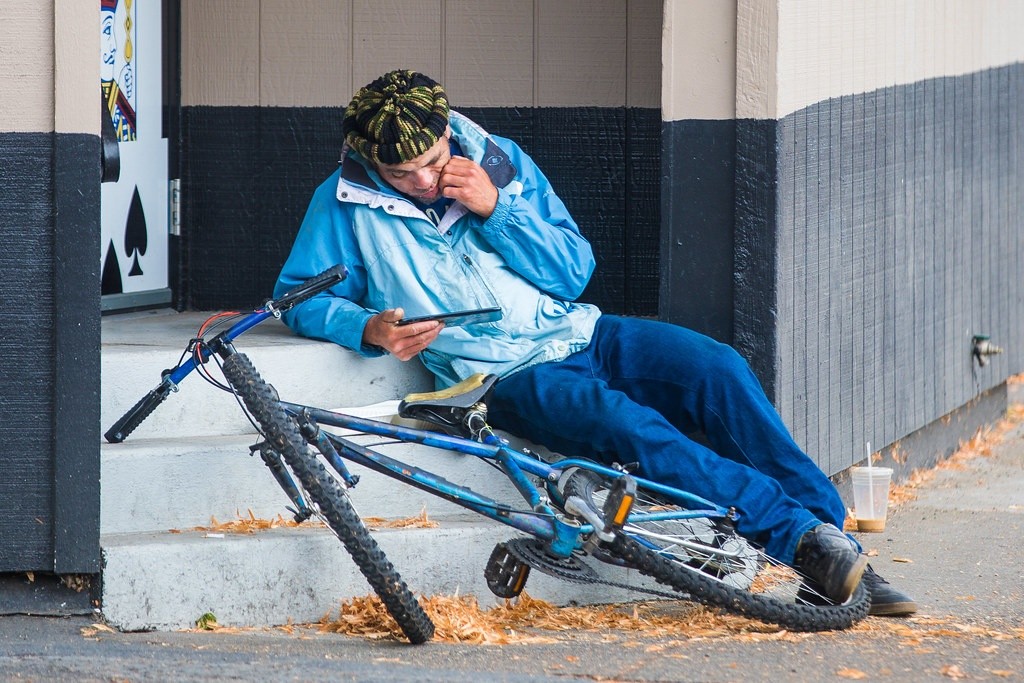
[794,523,867,603]
[795,563,918,616]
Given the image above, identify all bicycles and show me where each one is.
[105,265,873,645]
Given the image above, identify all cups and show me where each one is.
[850,465,894,535]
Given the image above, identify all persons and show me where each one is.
[272,67,919,617]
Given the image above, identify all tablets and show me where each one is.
[395,307,504,329]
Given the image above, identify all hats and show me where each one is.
[342,70,450,166]
